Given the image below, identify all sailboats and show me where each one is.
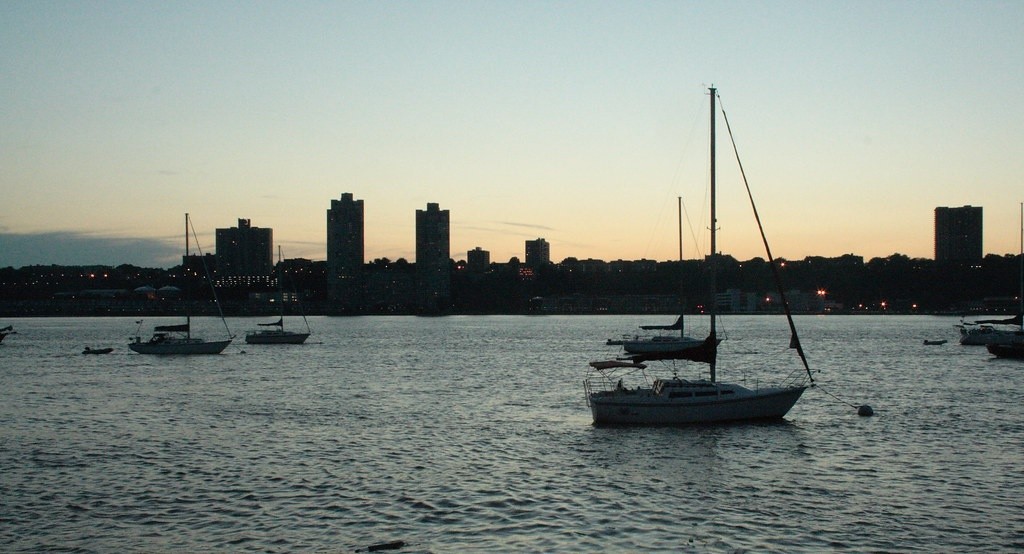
[127,212,237,356]
[581,83,816,427]
[621,193,725,356]
[958,203,1024,358]
[243,246,312,344]
